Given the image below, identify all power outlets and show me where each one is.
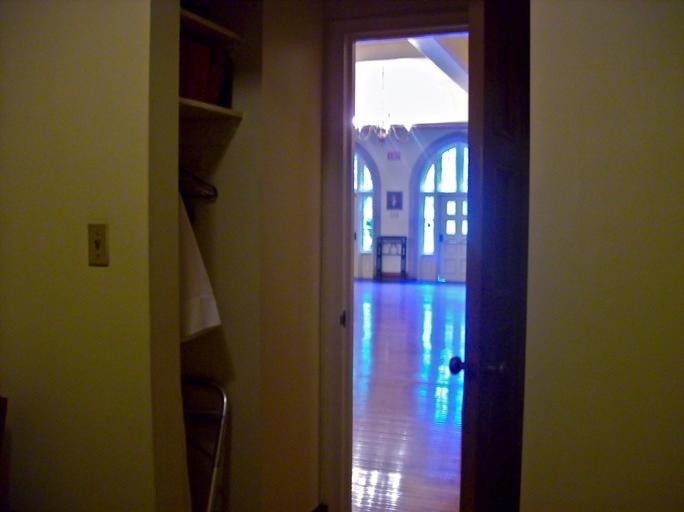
[86,222,109,267]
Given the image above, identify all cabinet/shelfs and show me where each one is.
[179,5,245,119]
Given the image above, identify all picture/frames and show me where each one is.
[386,192,403,209]
